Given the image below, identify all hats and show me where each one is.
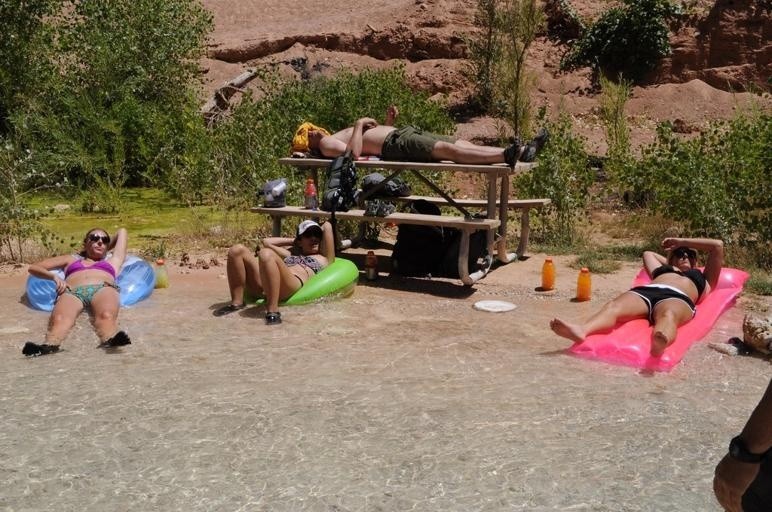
[297,220,323,237]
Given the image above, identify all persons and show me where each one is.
[551,238,723,357]
[21,226,132,354]
[309,106,545,166]
[712,381,772,510]
[214,219,335,322]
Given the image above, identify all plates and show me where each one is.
[474,300,517,313]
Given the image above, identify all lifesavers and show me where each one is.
[243,257,359,304]
[27,254,154,312]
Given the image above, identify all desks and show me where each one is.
[279,157,539,255]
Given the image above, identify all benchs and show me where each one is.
[250,205,501,286]
[398,196,552,264]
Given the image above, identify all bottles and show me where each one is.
[304,178,318,210]
[576,267,592,302]
[153,259,169,288]
[364,250,378,280]
[540,258,555,292]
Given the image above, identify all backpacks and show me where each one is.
[361,173,411,196]
[320,156,357,211]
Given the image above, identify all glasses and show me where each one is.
[675,248,695,259]
[89,234,110,243]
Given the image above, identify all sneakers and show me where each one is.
[266,311,282,324]
[214,302,246,316]
[377,201,398,217]
[505,136,520,166]
[519,128,545,162]
[364,199,380,216]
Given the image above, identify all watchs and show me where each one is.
[728,435,768,462]
